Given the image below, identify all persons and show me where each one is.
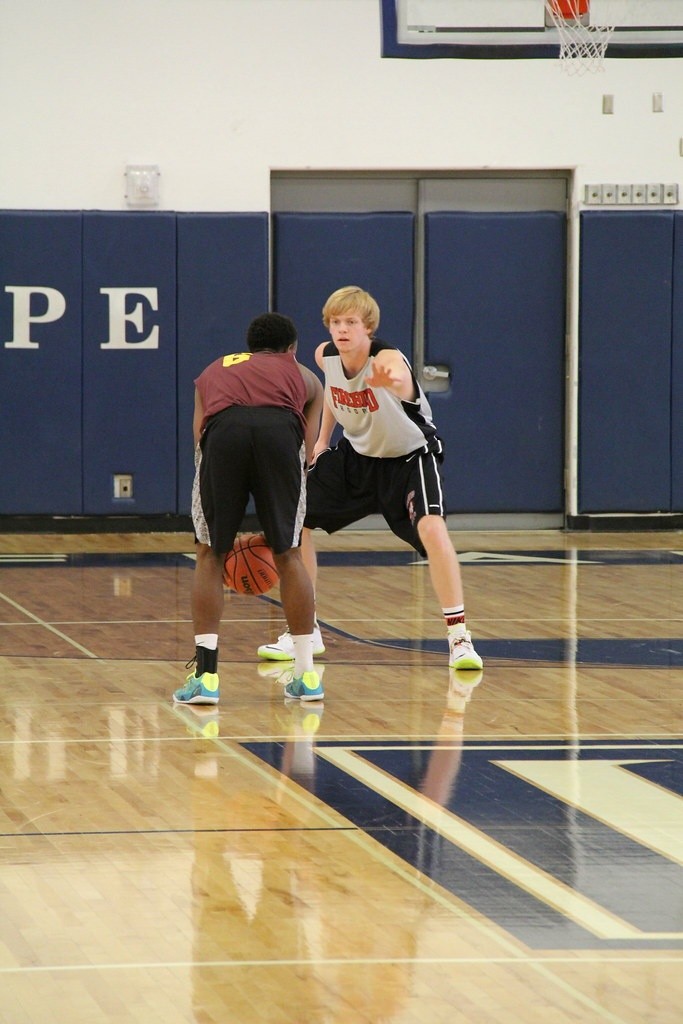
[256,286,484,671]
[171,314,326,704]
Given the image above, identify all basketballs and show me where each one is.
[221,534,281,598]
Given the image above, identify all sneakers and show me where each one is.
[257,625,326,660]
[447,631,483,669]
[285,672,325,701]
[172,667,220,703]
